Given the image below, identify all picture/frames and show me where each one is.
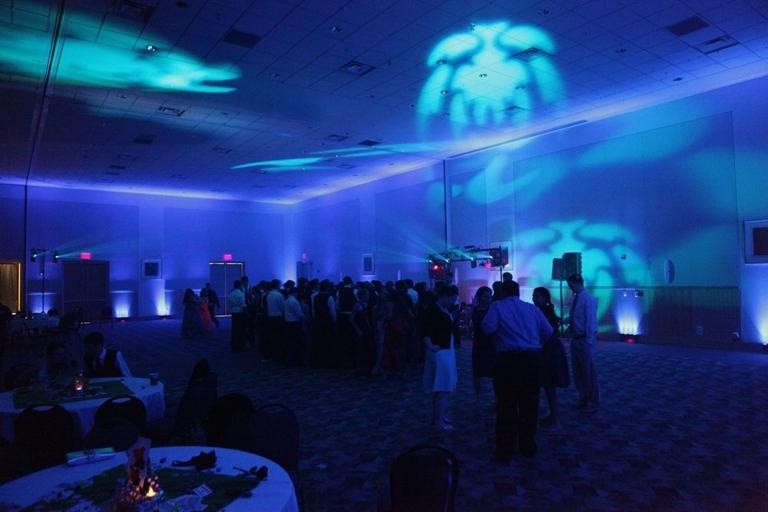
[141,258,162,279]
[361,253,375,274]
[743,219,768,265]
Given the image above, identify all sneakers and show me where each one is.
[540,401,599,426]
[432,416,456,433]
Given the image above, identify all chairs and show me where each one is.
[389,442,460,512]
[157,384,297,468]
[0,302,166,470]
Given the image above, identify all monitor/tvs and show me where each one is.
[141,258,162,279]
[744,218,768,264]
[361,254,375,275]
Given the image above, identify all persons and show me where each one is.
[419,286,458,435]
[471,285,497,399]
[503,273,512,282]
[200,283,221,329]
[491,281,502,299]
[531,286,565,427]
[82,332,133,378]
[229,279,251,351]
[199,290,216,334]
[240,275,462,376]
[481,280,554,463]
[567,273,600,414]
[183,288,209,339]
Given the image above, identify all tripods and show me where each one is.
[557,281,570,333]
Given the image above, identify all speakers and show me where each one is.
[552,258,567,280]
[489,247,509,266]
[562,252,582,279]
